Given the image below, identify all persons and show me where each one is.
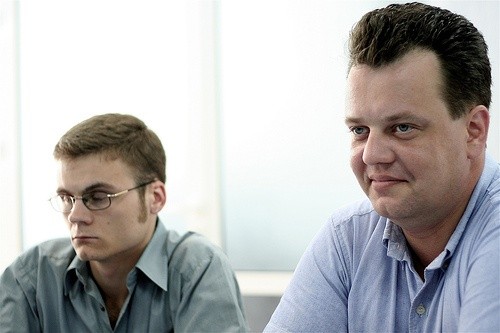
[259,1,498,333]
[0,113,249,333]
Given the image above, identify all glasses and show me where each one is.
[48,180,156,213]
